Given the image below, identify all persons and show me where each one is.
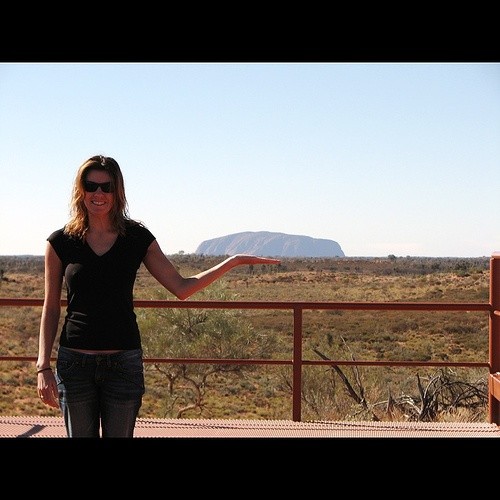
[34,153,284,438]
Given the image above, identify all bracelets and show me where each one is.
[36,367,53,376]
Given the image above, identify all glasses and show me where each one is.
[82,180,115,194]
